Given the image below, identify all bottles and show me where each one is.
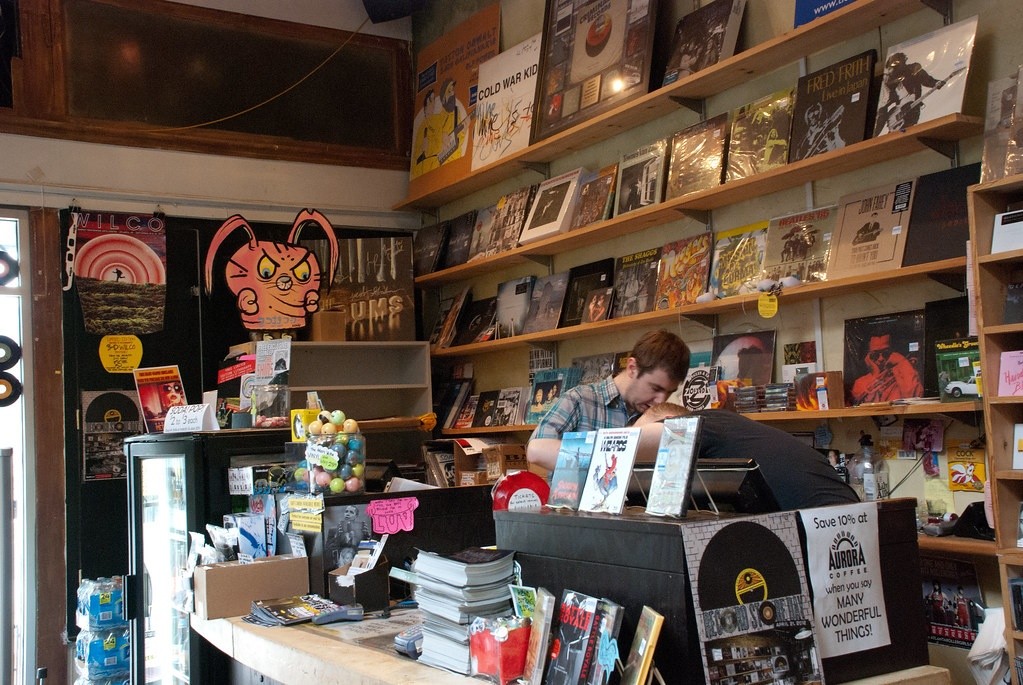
[250,384,291,428]
[848,433,889,502]
[74,576,130,685]
[307,433,366,495]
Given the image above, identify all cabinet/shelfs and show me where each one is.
[271,338,433,474]
[395,0,1023,685]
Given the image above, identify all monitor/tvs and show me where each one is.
[363,458,401,493]
[625,457,782,515]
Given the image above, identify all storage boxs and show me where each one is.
[192,554,311,621]
[491,497,930,684]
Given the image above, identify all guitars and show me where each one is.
[852,228,883,246]
[887,67,966,133]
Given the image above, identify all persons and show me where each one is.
[677,38,719,79]
[852,213,883,245]
[159,382,184,417]
[586,293,606,321]
[525,329,691,486]
[631,401,861,512]
[327,506,370,568]
[926,578,971,629]
[875,51,946,137]
[531,385,559,412]
[763,129,787,165]
[851,328,924,403]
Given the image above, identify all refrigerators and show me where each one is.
[124,428,308,685]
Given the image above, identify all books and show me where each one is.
[242,594,339,628]
